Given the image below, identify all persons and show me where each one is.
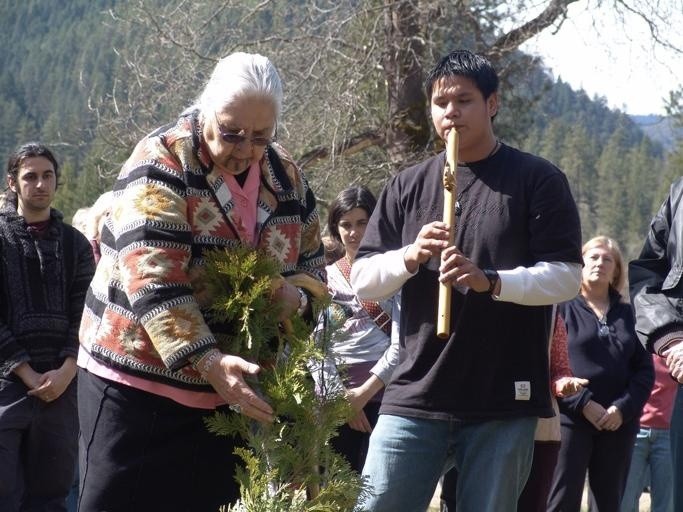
[71,51,327,510]
[1,140,95,511]
[348,50,583,510]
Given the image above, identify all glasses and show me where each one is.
[214,109,279,144]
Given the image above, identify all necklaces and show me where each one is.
[444,140,497,216]
